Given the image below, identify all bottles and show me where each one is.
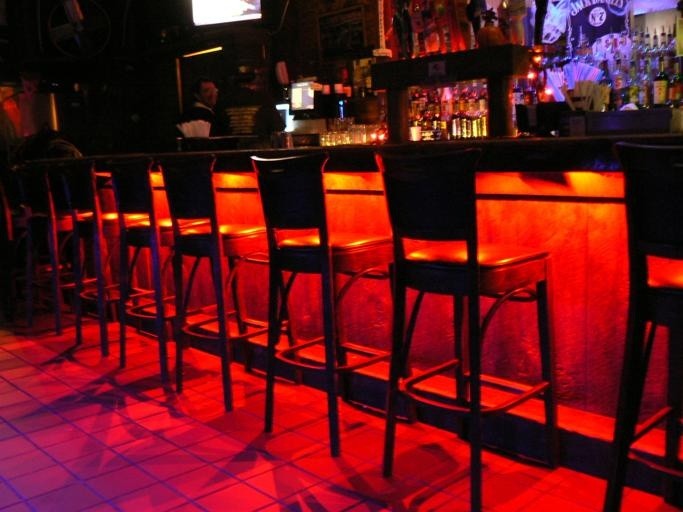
[409,80,486,142]
[352,59,373,99]
[518,29,682,108]
[390,0,476,59]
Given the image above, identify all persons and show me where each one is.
[175,79,218,140]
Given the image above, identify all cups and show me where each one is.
[317,120,386,146]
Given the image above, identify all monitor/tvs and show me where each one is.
[290,80,320,115]
[186,0,267,33]
